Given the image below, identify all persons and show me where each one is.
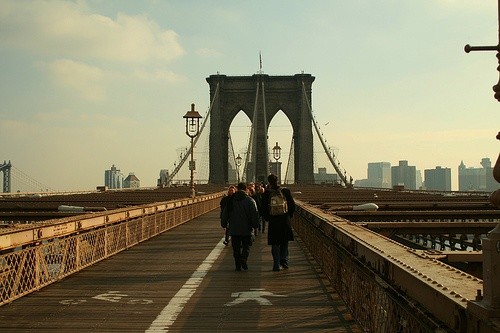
[221,182,259,272]
[223,174,296,271]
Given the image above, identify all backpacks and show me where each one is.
[268,187,289,216]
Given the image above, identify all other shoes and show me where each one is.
[273,266,282,271]
[223,241,229,246]
[240,257,248,269]
[235,268,241,271]
[282,262,289,269]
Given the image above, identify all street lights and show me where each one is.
[272,141,282,177]
[235,154,243,182]
[183,103,204,196]
